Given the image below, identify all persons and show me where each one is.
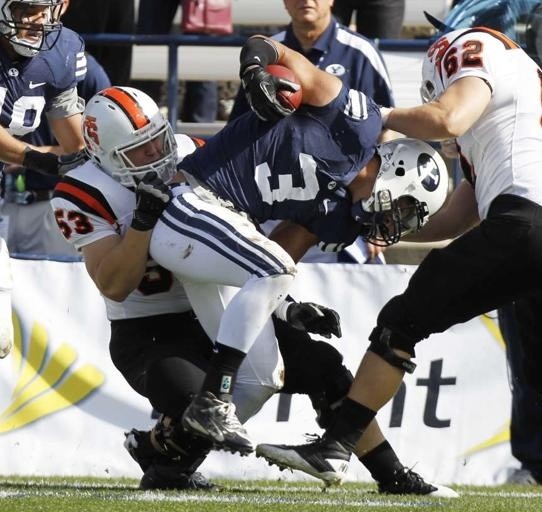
[124,35,450,497]
[51,86,461,498]
[1,0,113,262]
[229,0,395,265]
[63,1,218,121]
[255,27,542,487]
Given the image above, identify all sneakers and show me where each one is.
[378,463,460,499]
[125,429,168,474]
[181,391,255,458]
[140,464,225,492]
[255,432,352,488]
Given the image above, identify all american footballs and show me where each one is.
[263,64,302,111]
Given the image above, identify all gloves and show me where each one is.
[240,66,301,120]
[130,171,173,231]
[287,302,343,339]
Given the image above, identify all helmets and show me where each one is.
[82,86,177,189]
[0,0,70,56]
[352,137,449,248]
